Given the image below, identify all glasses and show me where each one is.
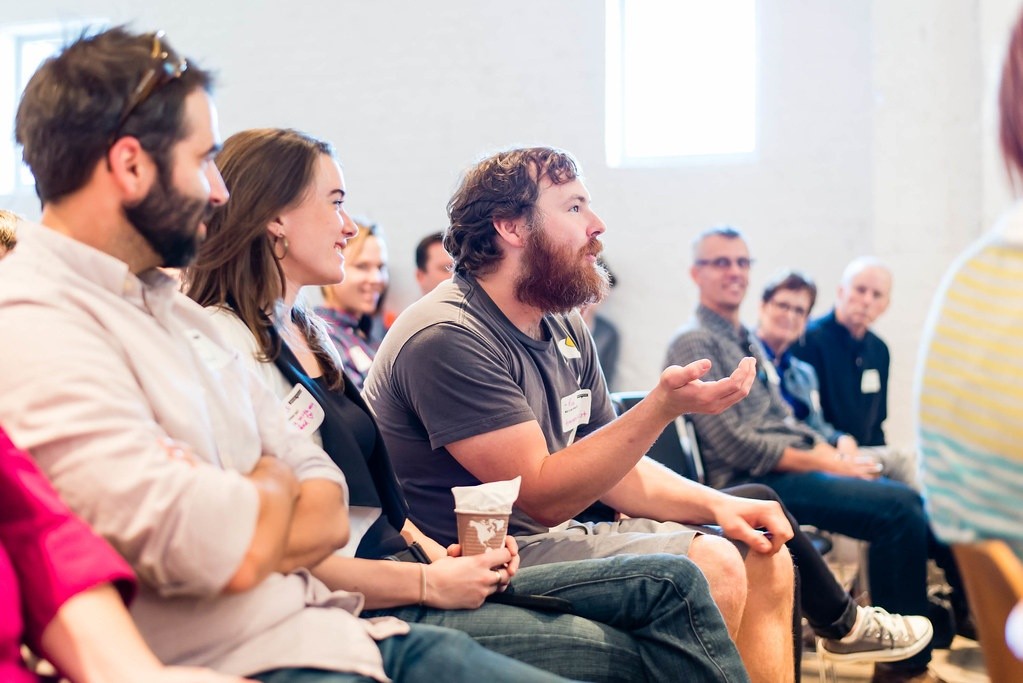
[695,258,750,271]
[117,31,186,133]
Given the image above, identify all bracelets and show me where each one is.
[417,561,427,606]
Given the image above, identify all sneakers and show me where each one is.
[818,605,933,663]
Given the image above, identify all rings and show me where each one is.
[494,568,502,584]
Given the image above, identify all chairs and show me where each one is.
[612,389,836,683]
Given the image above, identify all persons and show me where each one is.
[0,27,583,683]
[0,426,245,682]
[0,209,26,257]
[411,230,454,295]
[662,226,949,683]
[909,9,1023,566]
[310,217,388,386]
[361,143,799,683]
[190,126,758,682]
[753,249,980,649]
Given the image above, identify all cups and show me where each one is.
[452,506,515,563]
